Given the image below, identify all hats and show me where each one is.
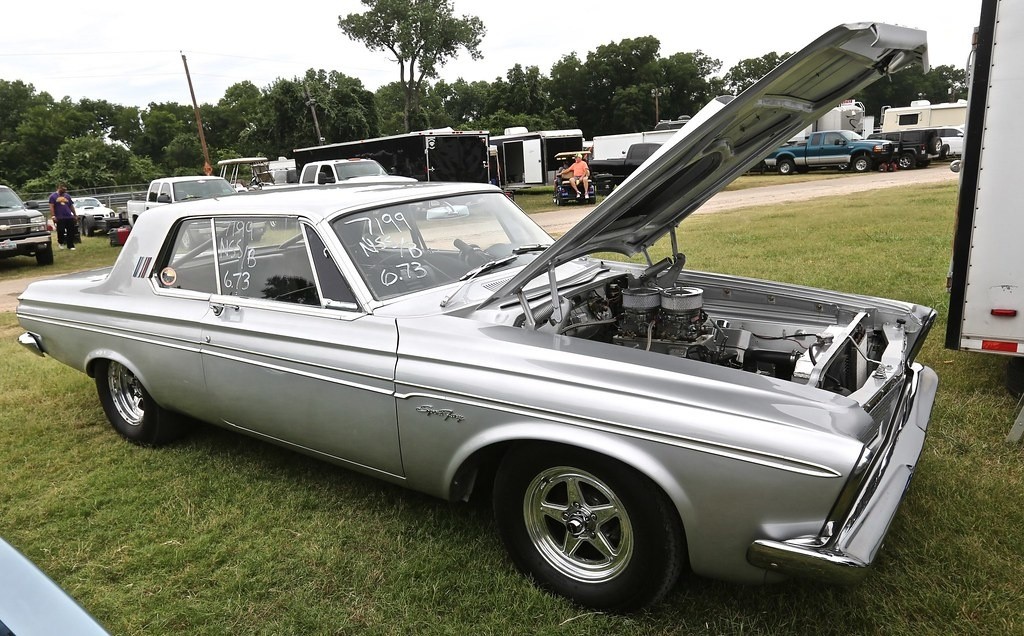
[574,153,583,158]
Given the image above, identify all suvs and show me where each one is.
[929,125,966,160]
[863,129,944,171]
[0,185,56,267]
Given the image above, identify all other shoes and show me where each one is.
[576,192,581,197]
[71,248,76,250]
[58,241,64,250]
[585,193,589,198]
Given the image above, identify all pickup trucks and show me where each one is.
[590,143,664,195]
[242,157,419,193]
[127,175,239,228]
[763,129,896,176]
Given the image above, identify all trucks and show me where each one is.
[783,99,865,146]
[941,0,1024,359]
[592,119,690,161]
[881,99,968,129]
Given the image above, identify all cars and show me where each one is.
[221,183,248,195]
[51,197,116,237]
[18,19,939,613]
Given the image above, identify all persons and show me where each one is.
[49,183,78,250]
[553,153,591,199]
